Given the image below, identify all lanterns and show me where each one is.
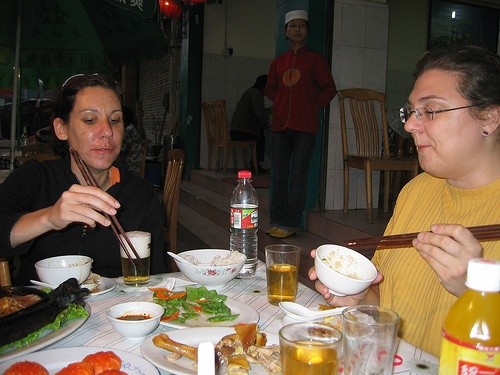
[157,0,206,20]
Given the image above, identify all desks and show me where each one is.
[383,107,420,208]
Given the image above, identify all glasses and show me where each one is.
[400,104,481,124]
[60,73,118,97]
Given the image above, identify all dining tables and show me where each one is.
[0,258,440,375]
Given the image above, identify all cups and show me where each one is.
[265,244,301,306]
[279,322,342,375]
[117,231,151,287]
[340,305,399,375]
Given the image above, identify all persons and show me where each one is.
[230,74,273,163]
[307,37,500,357]
[264,11,337,238]
[0,72,168,289]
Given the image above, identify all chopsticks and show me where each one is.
[344,224,500,250]
[69,146,145,269]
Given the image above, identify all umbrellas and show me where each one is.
[0,0,112,166]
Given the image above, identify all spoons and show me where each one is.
[31,279,97,292]
[278,300,350,321]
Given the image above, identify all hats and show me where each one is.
[285,10,309,25]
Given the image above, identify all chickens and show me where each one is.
[152,322,282,375]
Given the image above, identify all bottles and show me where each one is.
[436,257,500,375]
[228,170,258,279]
[18,126,31,152]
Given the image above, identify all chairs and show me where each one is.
[337,88,418,224]
[201,99,258,174]
[159,147,185,273]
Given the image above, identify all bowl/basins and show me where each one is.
[174,249,247,290]
[106,301,165,339]
[34,255,93,287]
[314,244,377,297]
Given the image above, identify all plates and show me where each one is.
[0,274,160,375]
[154,294,259,329]
[141,326,283,374]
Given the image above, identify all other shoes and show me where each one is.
[265,227,296,238]
[250,165,268,174]
